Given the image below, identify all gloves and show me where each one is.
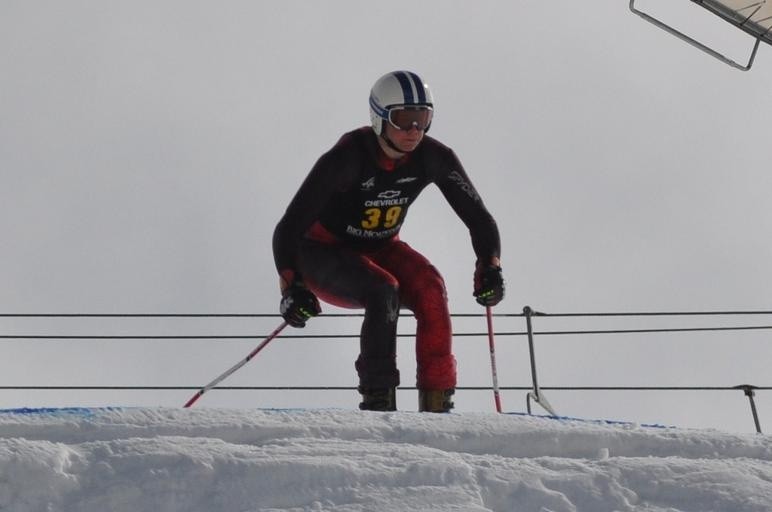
[280,286,321,328]
[473,263,504,306]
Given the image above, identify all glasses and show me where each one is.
[369,96,434,131]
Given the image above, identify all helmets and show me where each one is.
[369,71,434,136]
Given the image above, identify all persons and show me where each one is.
[272,69,506,413]
